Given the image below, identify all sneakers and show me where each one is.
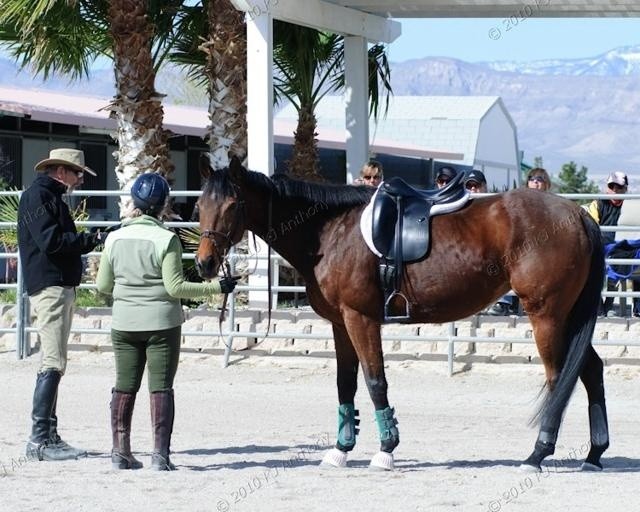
[487,302,508,315]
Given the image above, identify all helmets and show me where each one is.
[130,173,170,214]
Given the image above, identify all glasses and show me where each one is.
[437,179,449,183]
[528,176,544,182]
[68,166,84,179]
[363,175,379,180]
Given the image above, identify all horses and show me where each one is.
[195,154,609,472]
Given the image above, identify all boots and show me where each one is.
[149,389,179,471]
[50,387,87,459]
[109,387,144,469]
[25,371,77,462]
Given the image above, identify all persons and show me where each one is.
[96,173,241,470]
[435,166,457,189]
[464,170,487,193]
[17,148,109,461]
[354,161,383,186]
[477,168,550,316]
[586,171,640,319]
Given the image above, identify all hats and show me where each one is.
[435,166,457,179]
[465,170,486,185]
[606,171,628,186]
[33,147,97,178]
